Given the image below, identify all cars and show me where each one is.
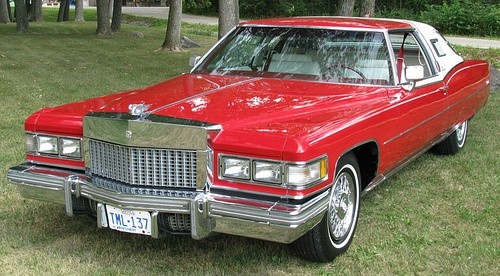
[7,16,491,264]
